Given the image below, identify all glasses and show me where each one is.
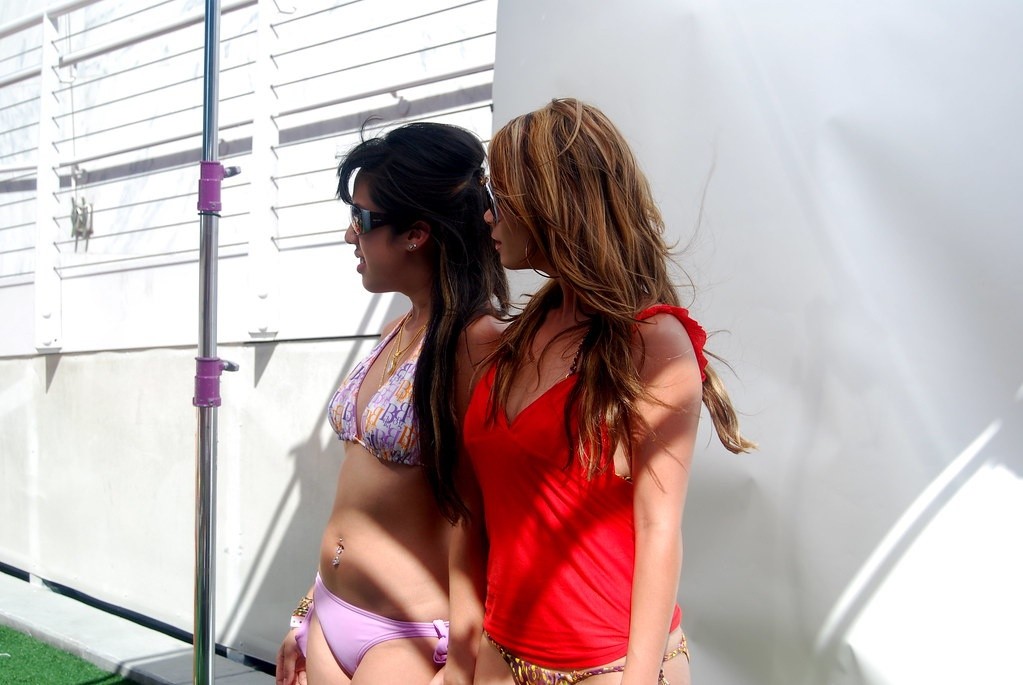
[351,203,420,235]
[486,182,509,226]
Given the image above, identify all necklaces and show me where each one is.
[378,313,430,389]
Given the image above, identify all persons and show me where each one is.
[277,122,519,685]
[461,96,759,685]
